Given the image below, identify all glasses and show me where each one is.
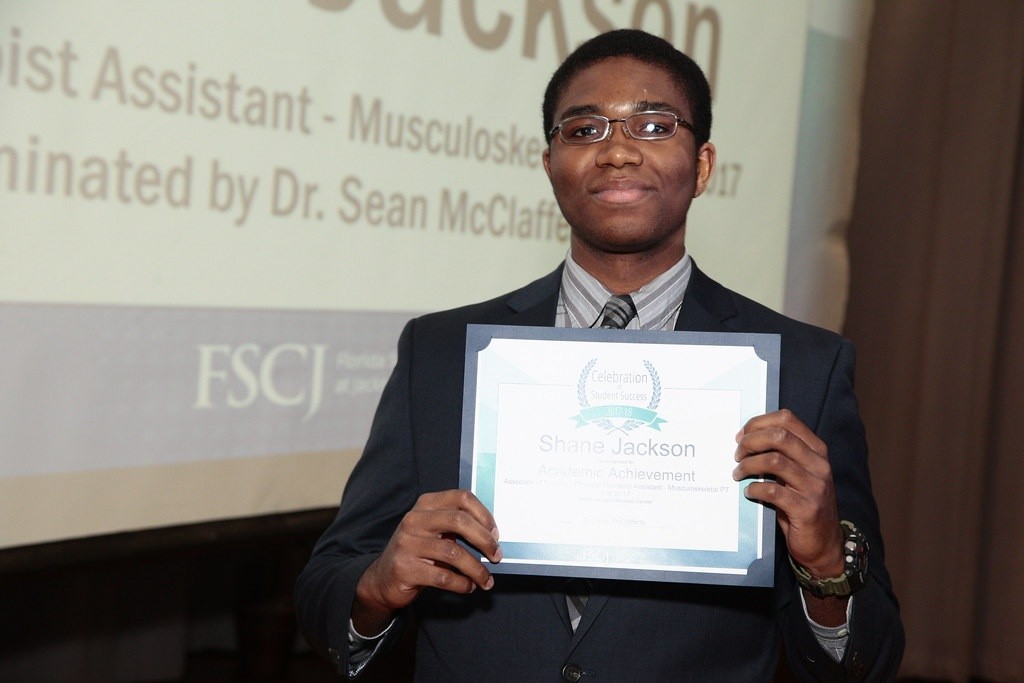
[551,110,695,146]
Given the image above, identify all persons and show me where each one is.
[294,29,908,683]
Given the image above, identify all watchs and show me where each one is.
[788,520,870,598]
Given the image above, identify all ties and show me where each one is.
[564,293,638,636]
